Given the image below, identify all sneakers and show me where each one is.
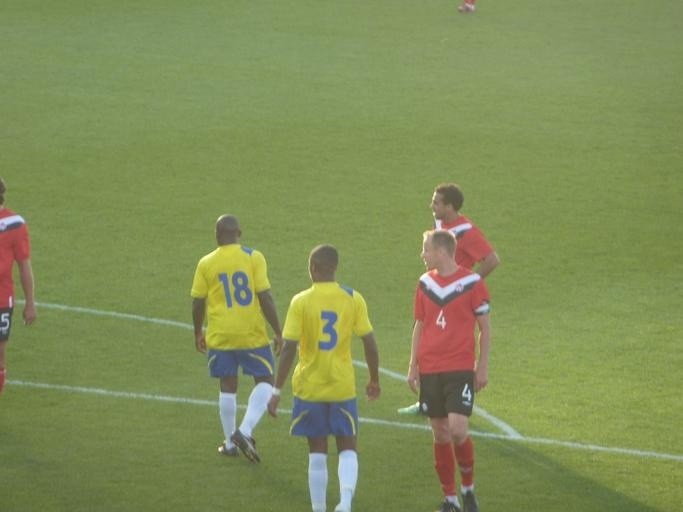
[440,500,459,511]
[231,428,260,464]
[218,441,237,456]
[397,401,427,416]
[462,487,479,512]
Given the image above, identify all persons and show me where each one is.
[190,213,283,465]
[407,229,491,511]
[1,178,36,392]
[395,184,500,418]
[266,243,382,512]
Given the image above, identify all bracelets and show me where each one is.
[270,386,281,395]
